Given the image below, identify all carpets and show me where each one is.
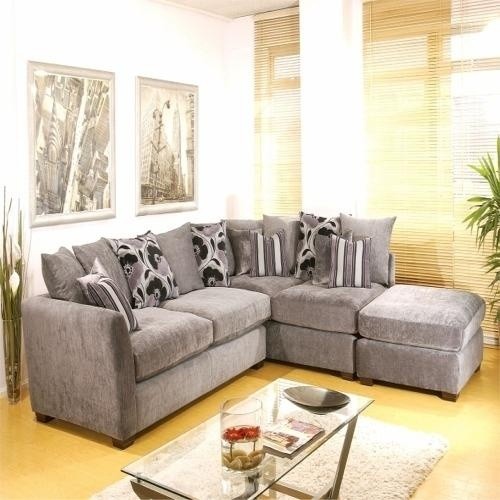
[94,414,449,498]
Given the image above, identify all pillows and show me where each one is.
[75,258,110,305]
[41,247,85,303]
[221,218,263,235]
[73,239,133,309]
[229,228,263,276]
[328,234,372,290]
[137,222,204,294]
[312,231,353,286]
[340,212,397,285]
[0,186,30,406]
[109,230,179,310]
[262,214,300,277]
[87,276,141,333]
[191,220,231,287]
[294,210,352,279]
[249,229,286,277]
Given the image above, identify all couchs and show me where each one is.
[227,252,395,382]
[22,285,270,450]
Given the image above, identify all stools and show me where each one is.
[355,285,486,401]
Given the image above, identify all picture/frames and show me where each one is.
[27,61,116,229]
[135,75,199,216]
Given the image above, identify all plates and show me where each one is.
[283,386,350,414]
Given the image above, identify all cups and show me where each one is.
[222,398,264,473]
